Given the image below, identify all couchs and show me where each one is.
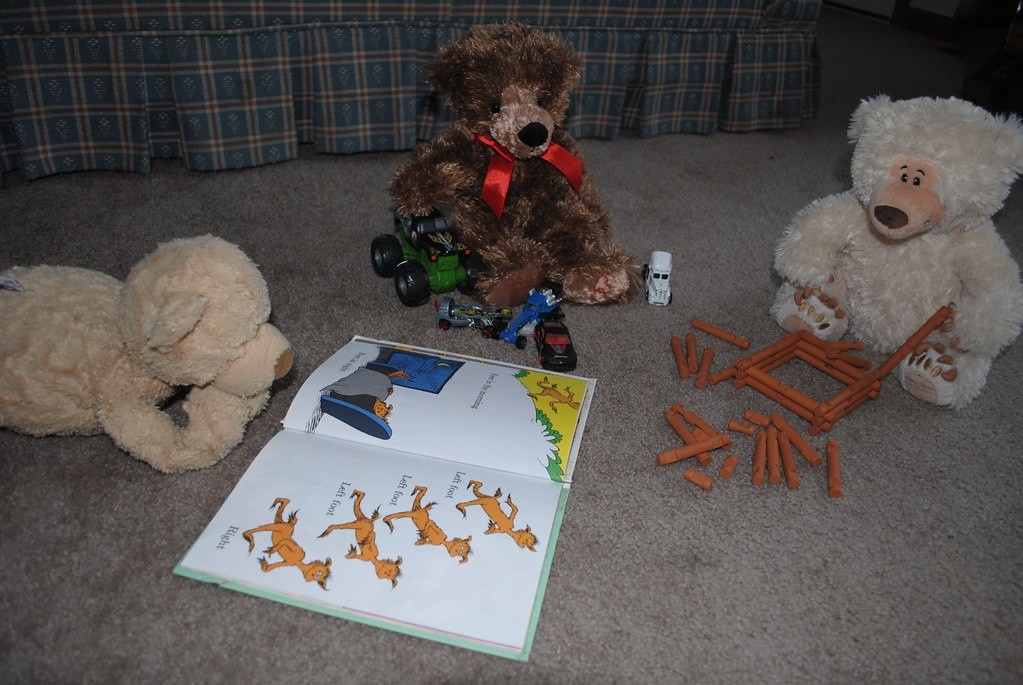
[0,1,827,196]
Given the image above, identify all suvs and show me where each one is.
[371,203,488,307]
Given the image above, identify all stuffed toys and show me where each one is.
[389,17,643,309]
[0,233,294,477]
[771,91,1023,411]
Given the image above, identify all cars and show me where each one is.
[642,250,673,307]
[533,321,577,373]
